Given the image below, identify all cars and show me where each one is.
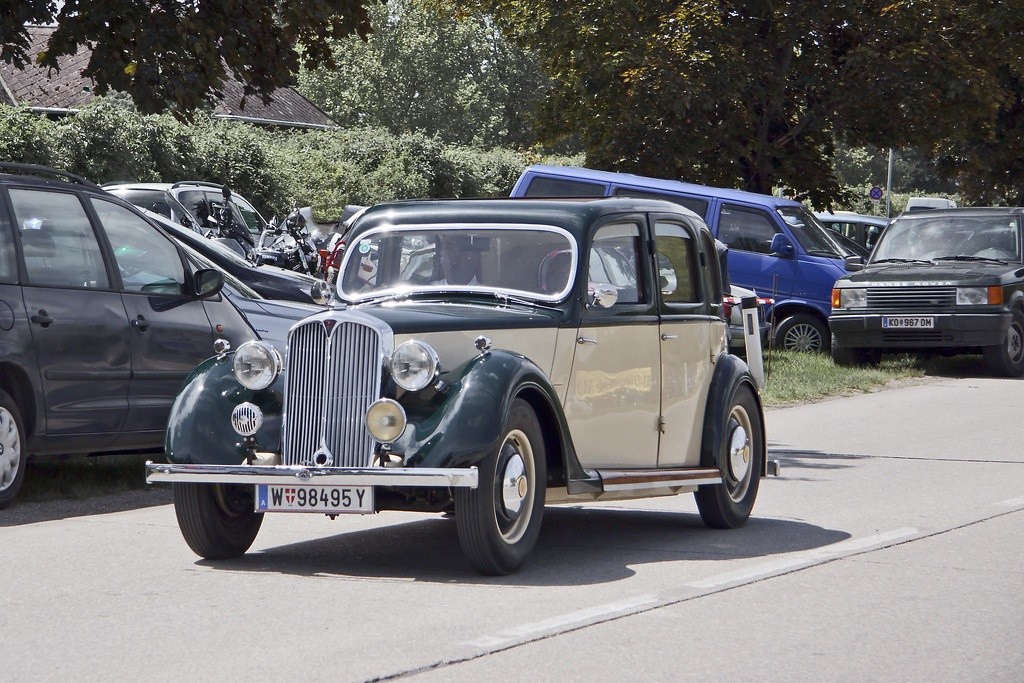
[145,194,783,576]
[773,196,1024,379]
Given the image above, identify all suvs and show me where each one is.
[0,159,381,510]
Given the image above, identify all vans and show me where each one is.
[510,163,861,362]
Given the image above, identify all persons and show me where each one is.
[427,234,482,286]
